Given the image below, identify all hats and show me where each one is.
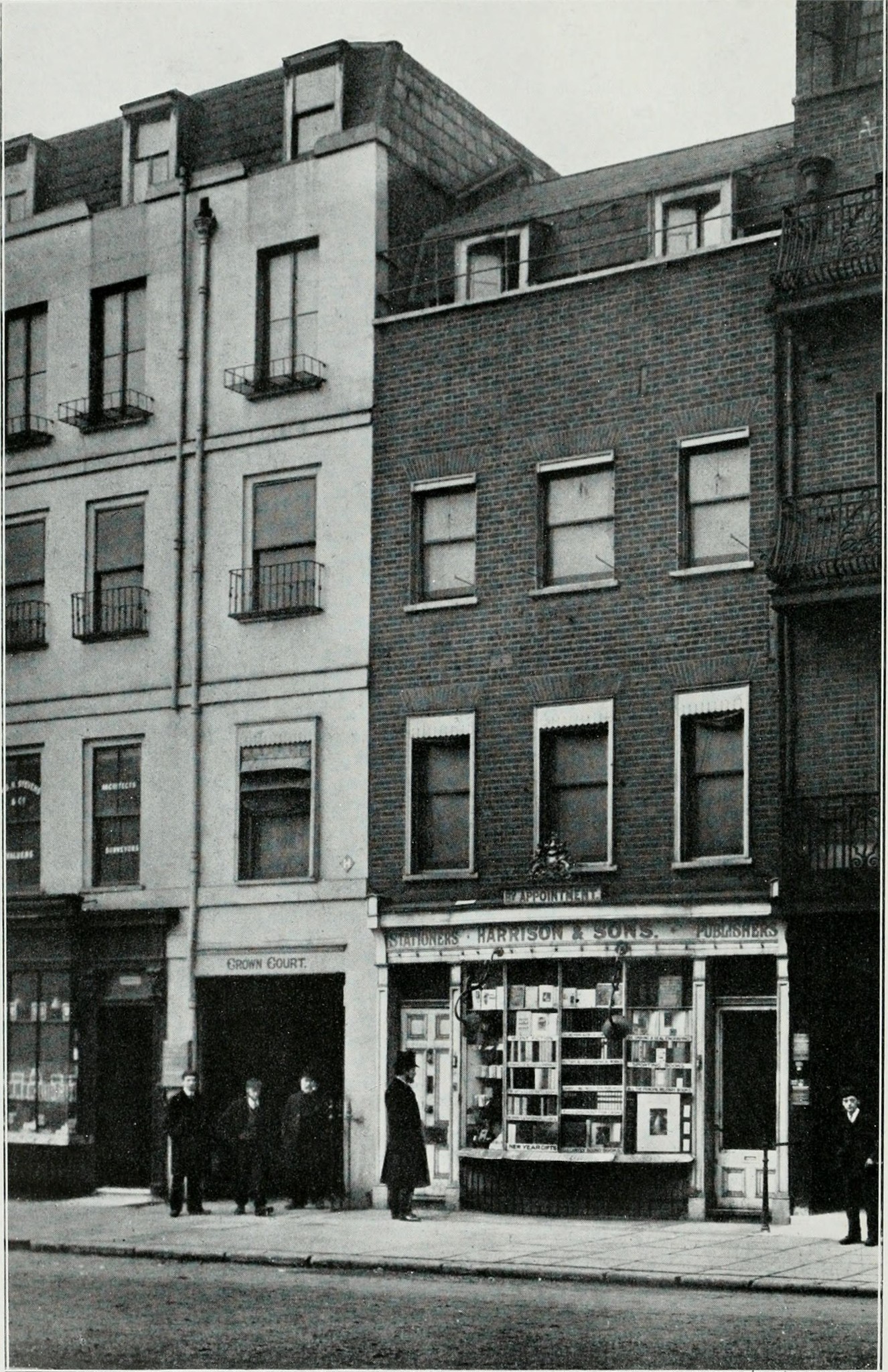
[841,1086,859,1099]
[395,1050,421,1069]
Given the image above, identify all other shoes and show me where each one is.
[171,1209,180,1217]
[393,1213,402,1219]
[189,1209,211,1215]
[234,1207,245,1215]
[316,1200,324,1209]
[255,1207,274,1215]
[332,1202,344,1211]
[285,1201,305,1209]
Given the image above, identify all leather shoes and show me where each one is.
[866,1238,877,1246]
[839,1237,861,1244]
[400,1213,420,1221]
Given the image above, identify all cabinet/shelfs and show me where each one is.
[459,975,695,1155]
[6,969,82,1137]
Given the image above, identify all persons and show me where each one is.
[162,1071,216,1216]
[831,1085,878,1247]
[280,1069,336,1208]
[217,1078,275,1215]
[377,1049,430,1221]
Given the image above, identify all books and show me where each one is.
[509,1067,556,1089]
[630,1040,689,1063]
[507,1095,556,1116]
[516,1011,559,1035]
[596,1091,622,1109]
[627,1068,689,1088]
[630,1009,689,1037]
[470,983,624,1010]
[510,1040,552,1063]
[600,1038,613,1059]
